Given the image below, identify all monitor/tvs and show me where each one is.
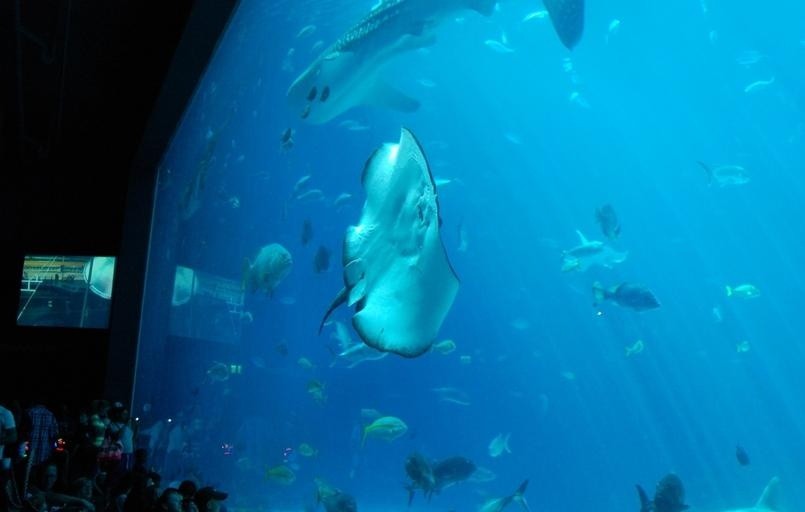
[163,264,249,348]
[15,254,119,330]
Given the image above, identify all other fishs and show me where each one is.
[166,1,800,512]
